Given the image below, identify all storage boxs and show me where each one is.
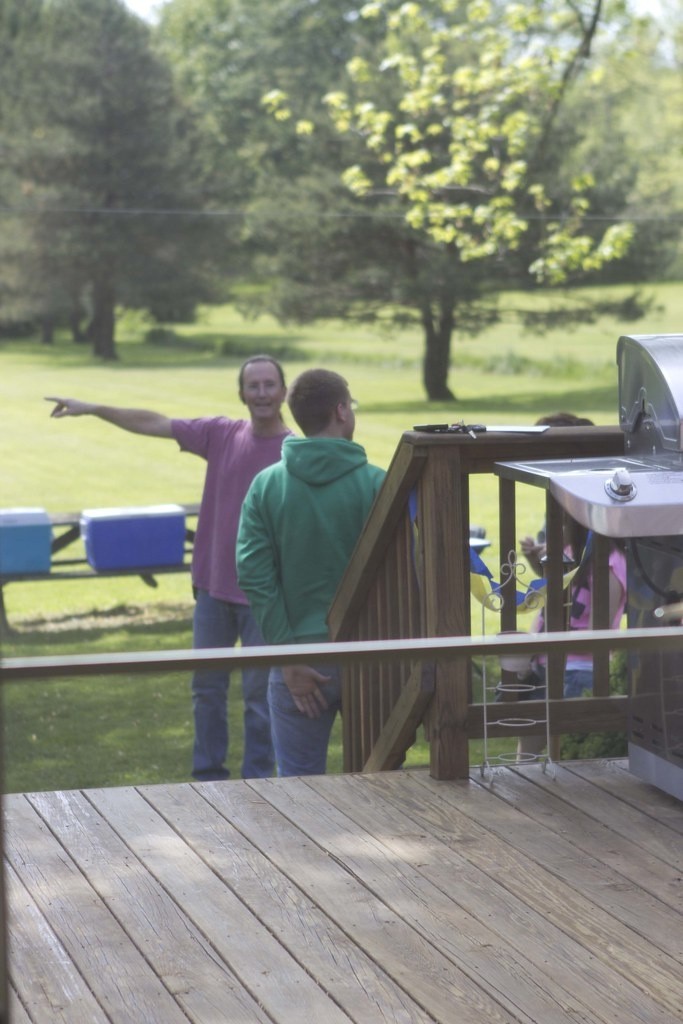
[80,504,187,573]
[0,508,51,574]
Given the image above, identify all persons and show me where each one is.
[236,369,415,778]
[518,411,629,764]
[44,355,298,783]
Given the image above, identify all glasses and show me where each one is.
[347,399,360,410]
